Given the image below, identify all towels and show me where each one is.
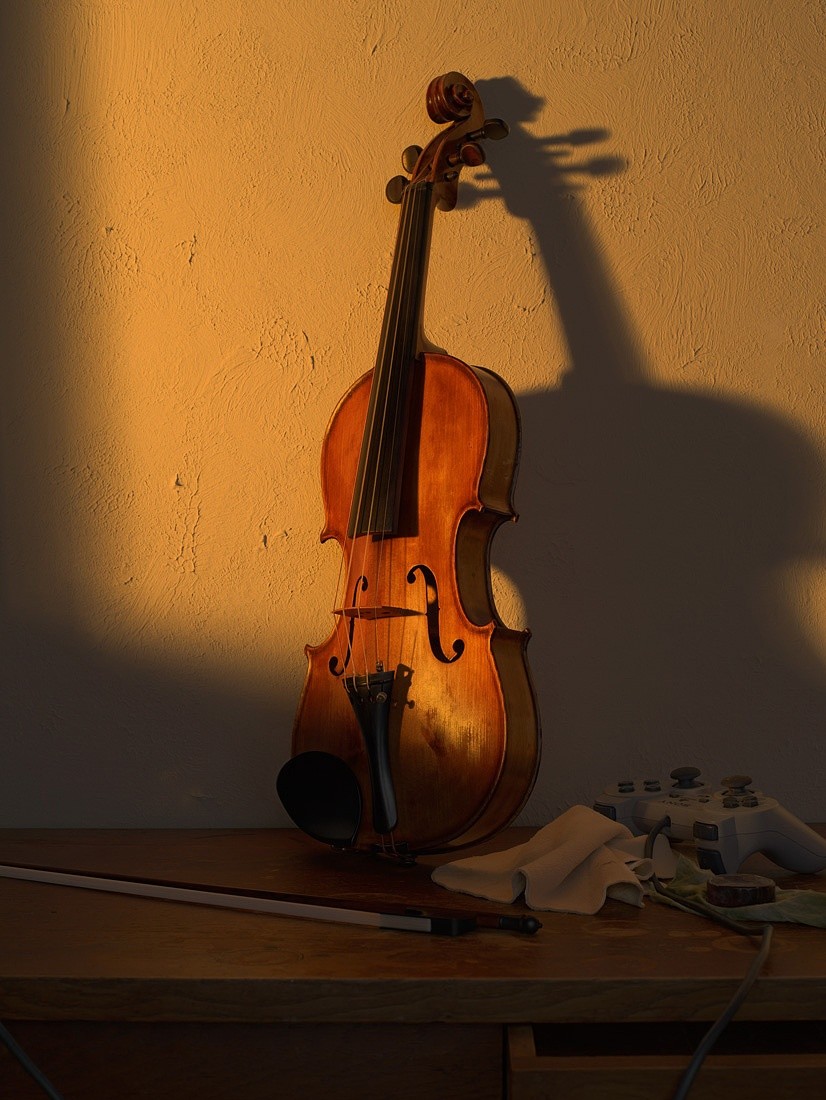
[647,848,826,929]
[428,805,659,915]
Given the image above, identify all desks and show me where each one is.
[0,824,826,1100]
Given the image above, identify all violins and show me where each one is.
[276,70,543,858]
